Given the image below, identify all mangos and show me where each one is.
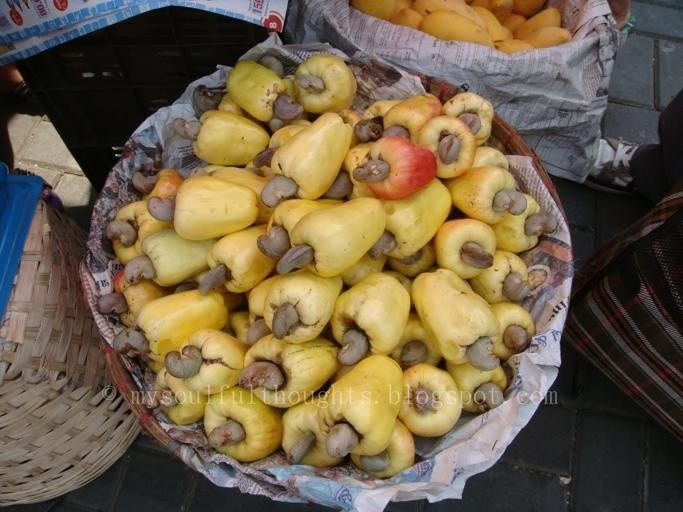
[351,1,573,54]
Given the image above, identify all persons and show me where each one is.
[583,87,683,199]
